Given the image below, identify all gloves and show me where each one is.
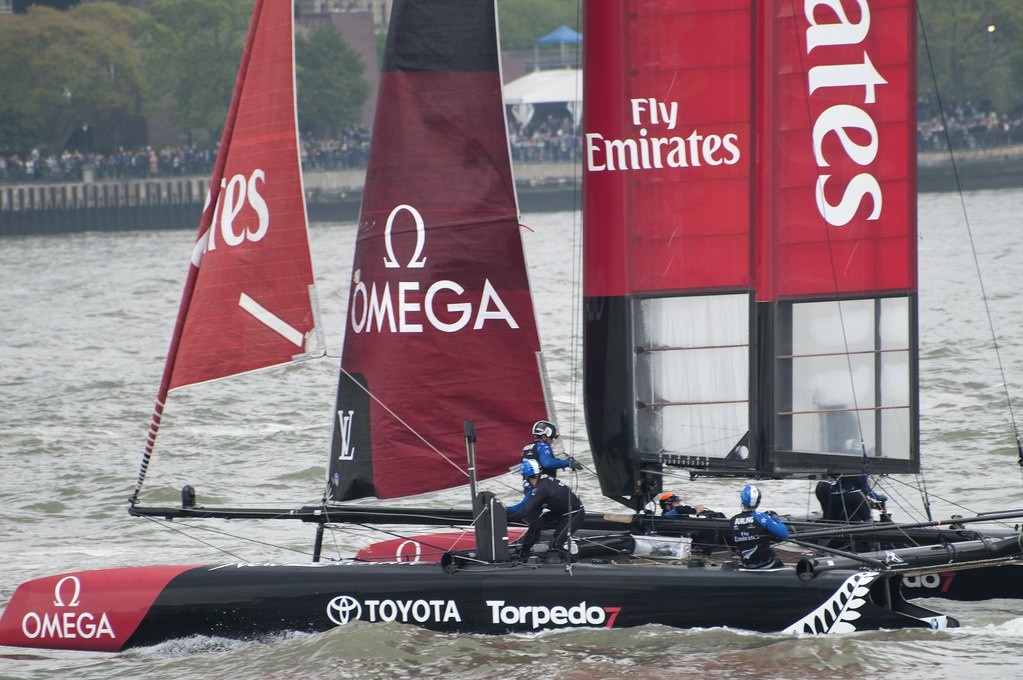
[764,510,777,519]
[571,462,583,471]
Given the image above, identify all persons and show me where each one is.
[813,406,888,522]
[0,143,231,185]
[506,459,586,562]
[508,116,584,163]
[298,123,370,172]
[914,88,1023,150]
[659,491,728,556]
[516,419,583,563]
[729,485,789,569]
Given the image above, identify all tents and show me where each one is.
[534,25,582,71]
[501,68,582,106]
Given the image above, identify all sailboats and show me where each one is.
[0,0,960,658]
[341,0,1023,601]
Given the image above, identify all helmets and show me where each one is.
[740,485,761,508]
[520,459,542,481]
[659,493,681,509]
[532,421,559,439]
